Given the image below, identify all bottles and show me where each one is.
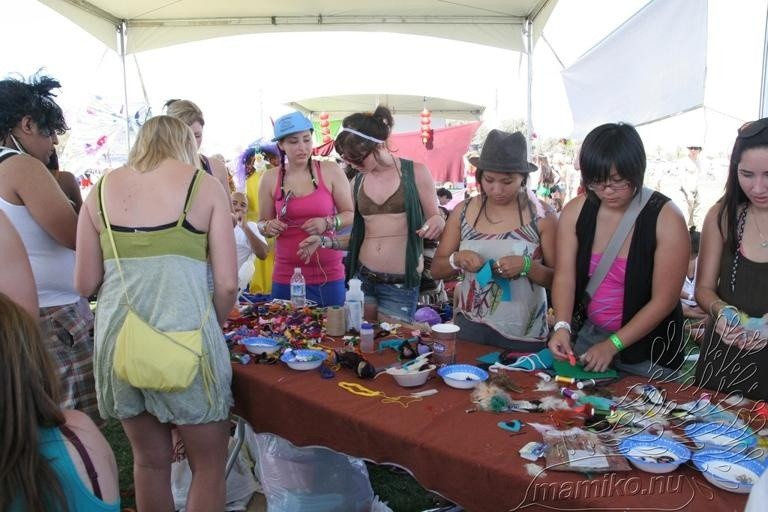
[346,279,364,321]
[360,322,375,353]
[291,267,306,310]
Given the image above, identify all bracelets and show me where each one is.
[554,320,572,334]
[325,216,330,231]
[330,216,336,234]
[609,333,624,351]
[263,221,269,235]
[335,216,340,233]
[449,251,462,271]
[330,236,337,249]
[717,305,739,319]
[709,299,727,319]
[520,254,533,276]
[320,235,325,248]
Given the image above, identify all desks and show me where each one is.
[219,299,768,512]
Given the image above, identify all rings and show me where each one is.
[495,258,502,264]
[421,224,429,231]
[497,266,503,274]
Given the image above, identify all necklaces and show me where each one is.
[750,203,768,246]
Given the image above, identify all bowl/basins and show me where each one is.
[385,364,437,388]
[438,363,489,390]
[241,337,283,356]
[692,448,767,494]
[684,422,759,452]
[619,434,691,474]
[279,348,328,370]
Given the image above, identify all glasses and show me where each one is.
[735,117,768,142]
[279,189,299,227]
[583,182,631,192]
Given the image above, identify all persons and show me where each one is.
[693,116,768,403]
[0,68,108,431]
[678,223,710,321]
[675,141,714,259]
[529,151,563,206]
[45,150,83,216]
[73,115,238,510]
[463,144,481,199]
[431,129,559,354]
[257,111,354,308]
[435,187,452,207]
[162,99,238,231]
[232,192,270,292]
[297,106,445,327]
[548,120,691,381]
[0,291,121,512]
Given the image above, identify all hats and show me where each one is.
[466,128,538,174]
[269,110,315,142]
